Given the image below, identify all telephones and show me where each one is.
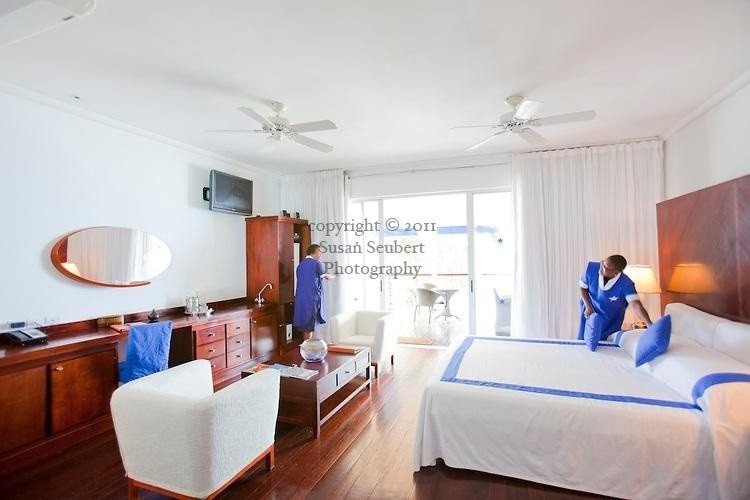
[0,328,48,347]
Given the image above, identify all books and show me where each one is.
[110,321,150,333]
[326,343,366,355]
[266,362,320,381]
[240,361,272,376]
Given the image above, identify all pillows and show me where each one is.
[583,310,600,351]
[613,326,750,425]
[633,315,671,368]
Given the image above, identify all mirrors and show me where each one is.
[51,225,173,287]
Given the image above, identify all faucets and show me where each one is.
[254,283,273,303]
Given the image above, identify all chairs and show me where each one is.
[118,320,173,385]
[413,289,440,325]
[330,309,398,379]
[110,356,282,500]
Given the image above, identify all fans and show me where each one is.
[204,101,340,155]
[450,96,596,153]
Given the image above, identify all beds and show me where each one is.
[413,303,749,500]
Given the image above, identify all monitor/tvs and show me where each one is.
[210,168,254,217]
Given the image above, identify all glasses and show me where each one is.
[600,260,617,271]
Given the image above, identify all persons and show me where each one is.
[577,254,654,342]
[294,242,338,342]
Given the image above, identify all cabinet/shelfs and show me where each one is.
[1,348,118,456]
[245,214,312,359]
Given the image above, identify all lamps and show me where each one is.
[667,263,720,294]
[623,263,662,327]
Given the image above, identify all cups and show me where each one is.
[185,293,208,313]
